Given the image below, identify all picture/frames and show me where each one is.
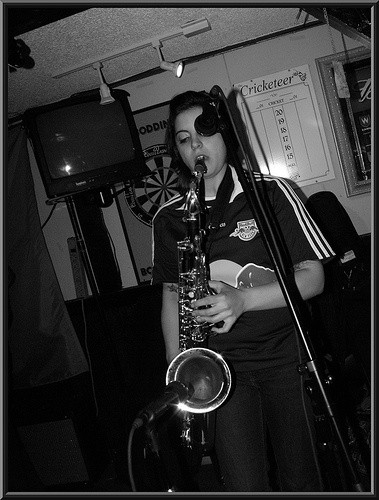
[315,45,371,198]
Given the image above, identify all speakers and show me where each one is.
[13,417,91,488]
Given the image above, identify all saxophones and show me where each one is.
[166,157,233,457]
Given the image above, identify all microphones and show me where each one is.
[194,83,220,137]
[132,379,189,430]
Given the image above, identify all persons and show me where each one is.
[151,91,336,491]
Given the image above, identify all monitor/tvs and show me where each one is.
[23,89,147,200]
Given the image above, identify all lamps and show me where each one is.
[152,41,183,77]
[92,61,115,105]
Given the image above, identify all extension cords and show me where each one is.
[67,236,88,298]
